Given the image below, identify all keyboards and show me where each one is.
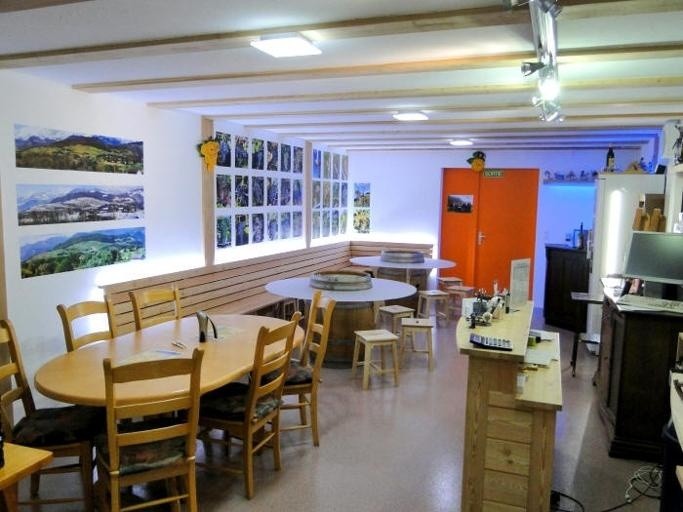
[617,293,683,314]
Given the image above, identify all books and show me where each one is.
[530,329,552,341]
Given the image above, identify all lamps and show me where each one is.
[250,32,322,59]
[450,138,474,146]
[512,0,565,123]
[392,110,429,120]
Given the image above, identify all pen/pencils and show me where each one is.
[474,288,492,303]
[172,341,187,349]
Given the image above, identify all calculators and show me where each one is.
[469,333,513,351]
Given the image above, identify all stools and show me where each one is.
[350,277,475,390]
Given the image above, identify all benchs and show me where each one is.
[104,241,433,335]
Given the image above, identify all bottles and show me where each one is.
[604,142,615,170]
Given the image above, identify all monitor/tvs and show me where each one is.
[622,231,683,285]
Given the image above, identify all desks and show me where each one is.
[265,276,417,369]
[570,291,625,378]
[350,257,457,316]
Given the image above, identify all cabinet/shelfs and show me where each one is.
[595,295,683,463]
